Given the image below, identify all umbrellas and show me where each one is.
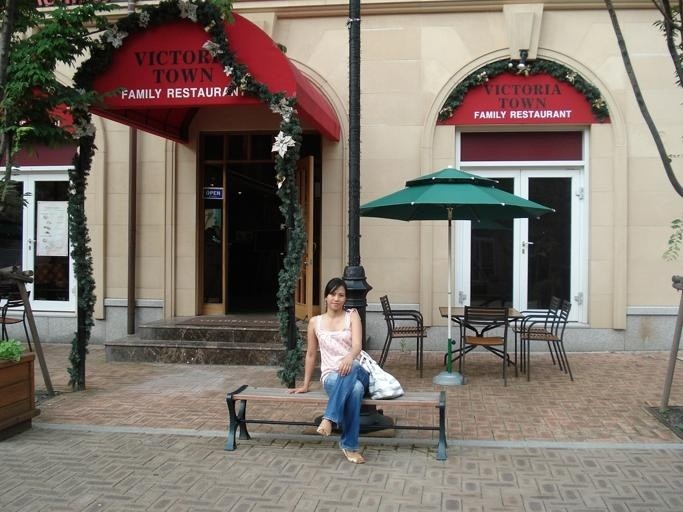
[357,164,559,373]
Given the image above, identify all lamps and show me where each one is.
[517,50,528,71]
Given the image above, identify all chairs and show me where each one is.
[458,305,509,386]
[0,291,32,351]
[511,295,562,371]
[378,295,428,378]
[521,300,573,382]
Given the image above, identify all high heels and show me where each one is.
[316,419,332,437]
[338,441,365,463]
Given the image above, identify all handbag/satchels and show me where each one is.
[358,348,405,401]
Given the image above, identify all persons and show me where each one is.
[286,277,371,465]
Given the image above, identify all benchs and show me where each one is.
[224,385,449,461]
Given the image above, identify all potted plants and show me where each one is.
[0,340,41,442]
